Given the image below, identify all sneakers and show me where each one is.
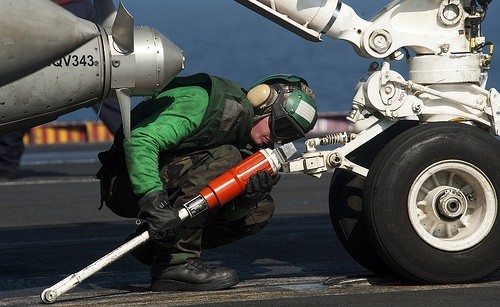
[124,227,157,266]
[151,255,240,291]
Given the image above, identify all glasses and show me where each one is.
[268,103,305,145]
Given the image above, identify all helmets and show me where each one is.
[246,73,318,134]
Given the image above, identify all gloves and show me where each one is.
[234,170,281,208]
[137,190,181,243]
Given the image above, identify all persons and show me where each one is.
[97,73,319,292]
